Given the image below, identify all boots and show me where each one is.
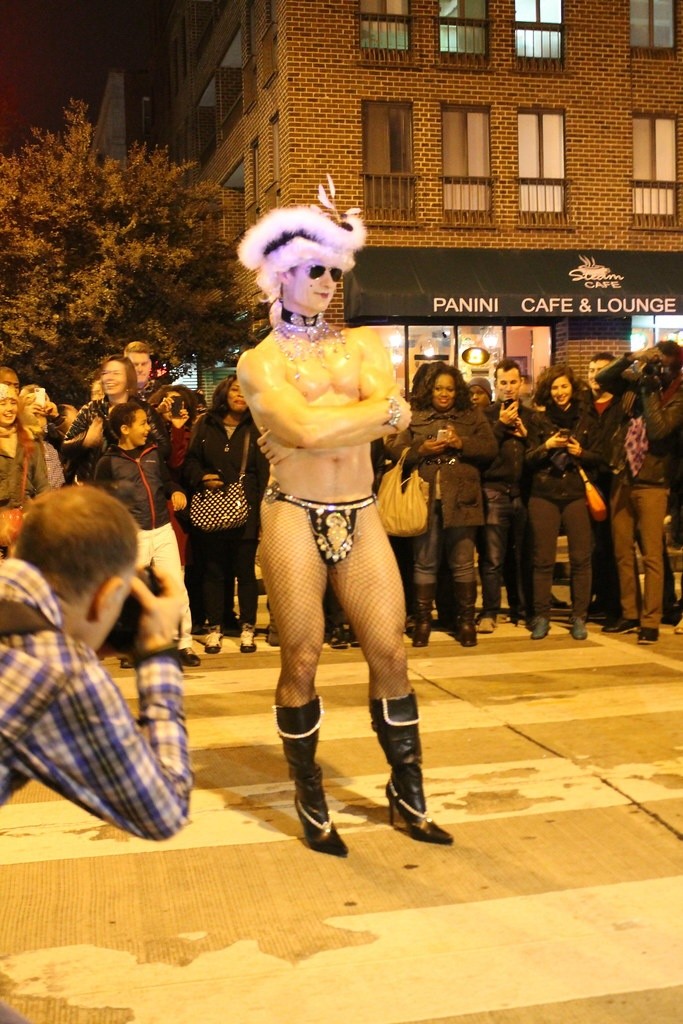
[274,696,350,858]
[454,581,477,646]
[413,582,436,646]
[367,686,455,845]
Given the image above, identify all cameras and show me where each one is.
[641,358,665,382]
[105,565,161,659]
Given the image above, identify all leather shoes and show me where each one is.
[329,626,361,647]
[638,626,660,643]
[602,616,639,635]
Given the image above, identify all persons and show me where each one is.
[0,340,683,653]
[235,207,454,855]
[0,483,193,841]
[94,403,202,668]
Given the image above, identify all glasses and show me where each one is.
[295,264,343,282]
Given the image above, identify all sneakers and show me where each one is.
[241,623,256,652]
[205,626,223,654]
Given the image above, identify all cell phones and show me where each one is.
[171,395,183,418]
[504,399,514,410]
[559,429,570,438]
[33,388,45,408]
[436,429,448,443]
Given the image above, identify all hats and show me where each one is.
[236,176,367,302]
[467,377,492,400]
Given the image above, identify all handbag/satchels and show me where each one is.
[583,481,608,521]
[0,507,24,546]
[189,480,248,529]
[376,449,428,536]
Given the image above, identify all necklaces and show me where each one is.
[273,308,351,379]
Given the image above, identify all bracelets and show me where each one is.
[386,396,401,426]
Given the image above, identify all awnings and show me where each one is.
[341,245,683,326]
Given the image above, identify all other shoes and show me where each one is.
[549,595,567,608]
[569,613,588,639]
[587,604,610,619]
[518,603,530,628]
[121,656,135,668]
[673,618,683,634]
[178,647,201,666]
[478,610,497,632]
[267,618,279,646]
[532,617,550,639]
